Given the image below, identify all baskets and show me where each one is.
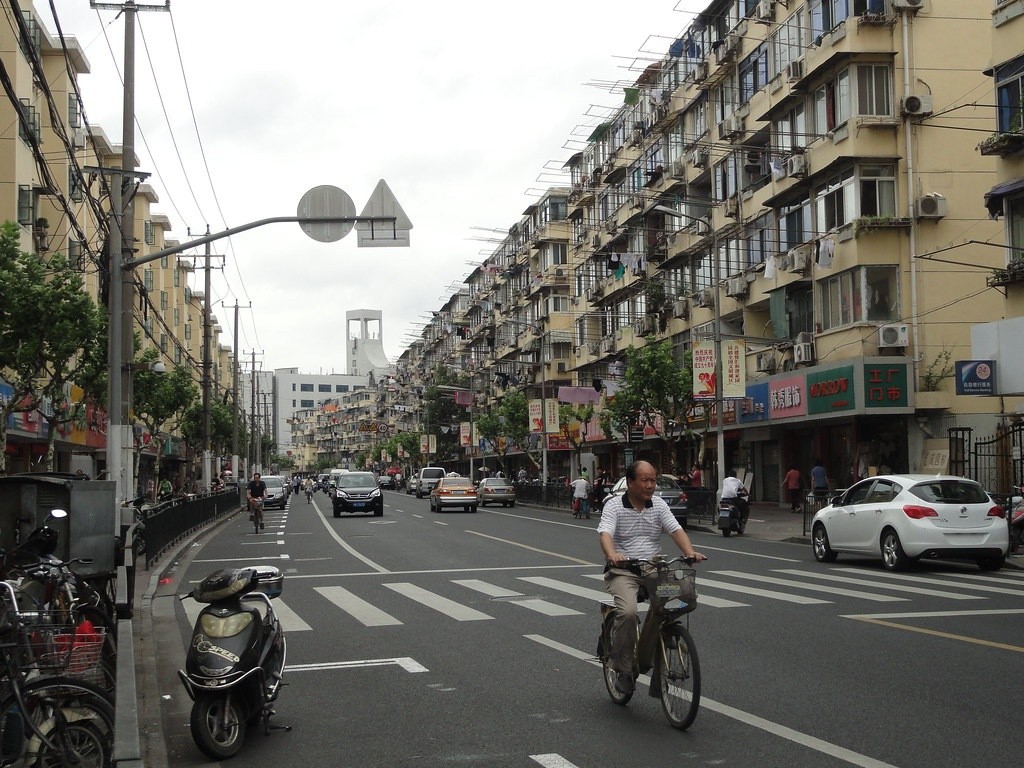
[642,568,699,614]
[8,608,107,696]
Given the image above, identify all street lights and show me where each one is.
[388,406,405,489]
[445,364,474,485]
[505,317,548,504]
[654,204,727,524]
[408,390,430,467]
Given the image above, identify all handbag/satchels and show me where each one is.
[572,497,591,514]
[805,489,816,506]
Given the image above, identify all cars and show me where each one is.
[376,475,394,490]
[809,471,1010,572]
[405,473,418,494]
[475,477,517,508]
[430,476,480,514]
[601,476,688,532]
[249,474,294,510]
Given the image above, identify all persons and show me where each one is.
[581,466,589,480]
[158,476,172,494]
[688,464,701,487]
[303,475,314,501]
[211,473,220,485]
[495,469,505,478]
[219,475,224,484]
[246,473,268,529]
[518,466,528,480]
[594,467,606,512]
[322,476,328,492]
[780,463,802,513]
[597,461,708,694]
[717,471,750,528]
[293,473,301,494]
[811,459,832,508]
[568,475,590,519]
[394,472,403,487]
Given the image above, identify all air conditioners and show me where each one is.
[726,114,742,136]
[877,324,909,349]
[793,343,812,364]
[784,250,808,274]
[785,61,800,82]
[916,197,948,221]
[893,0,924,13]
[786,154,804,179]
[725,277,746,297]
[714,44,728,67]
[75,131,87,150]
[717,119,728,141]
[744,149,762,168]
[724,35,737,55]
[898,94,934,117]
[722,198,737,217]
[755,0,772,25]
[462,64,714,406]
[754,350,776,373]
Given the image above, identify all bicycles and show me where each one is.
[302,483,314,504]
[581,554,709,732]
[248,497,265,534]
[0,506,118,768]
[568,484,592,519]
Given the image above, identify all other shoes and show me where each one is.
[797,507,801,513]
[790,508,794,513]
[661,633,677,650]
[614,670,635,696]
[260,523,265,529]
[249,514,255,521]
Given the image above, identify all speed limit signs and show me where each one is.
[378,423,388,433]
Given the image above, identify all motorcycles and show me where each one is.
[717,491,752,538]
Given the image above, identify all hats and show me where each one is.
[578,476,588,480]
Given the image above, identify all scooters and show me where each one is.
[125,490,174,556]
[175,564,291,760]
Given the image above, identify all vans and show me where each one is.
[413,467,447,500]
[300,468,351,497]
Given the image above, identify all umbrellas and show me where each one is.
[478,467,492,472]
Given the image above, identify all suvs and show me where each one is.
[329,471,384,518]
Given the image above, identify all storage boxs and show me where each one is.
[243,566,284,599]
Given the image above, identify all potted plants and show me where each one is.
[36,216,49,235]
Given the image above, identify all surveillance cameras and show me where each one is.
[154,363,166,375]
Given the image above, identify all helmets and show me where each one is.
[307,474,310,478]
